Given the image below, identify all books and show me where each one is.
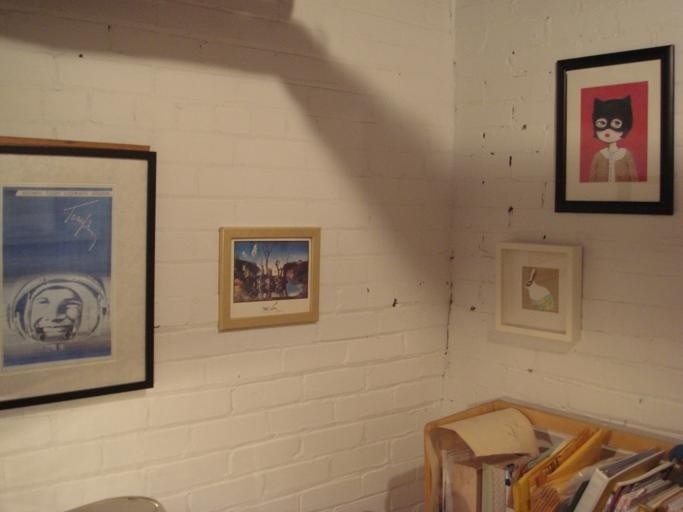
[431,417,682,511]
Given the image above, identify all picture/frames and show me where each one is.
[495,241,581,342]
[0,136,156,411]
[551,45,674,216]
[218,226,320,331]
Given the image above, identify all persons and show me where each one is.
[5,270,108,346]
